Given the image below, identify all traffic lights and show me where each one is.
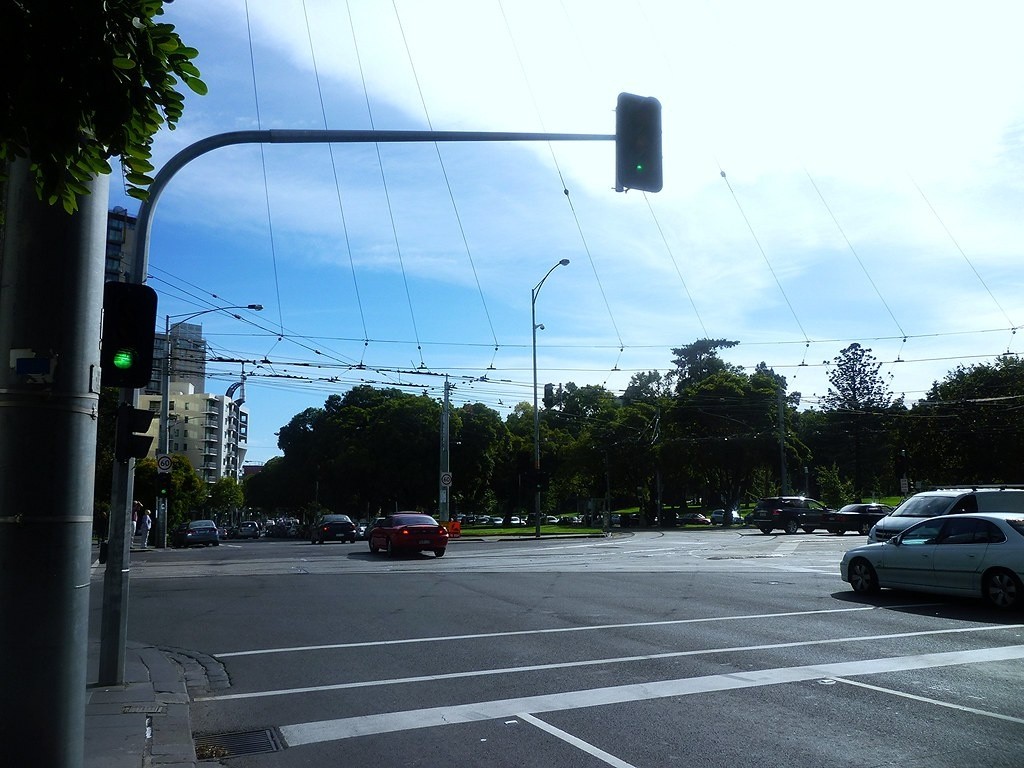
[115,401,155,463]
[101,282,158,388]
[157,472,172,497]
[615,90,663,193]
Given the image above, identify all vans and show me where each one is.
[865,484,1024,549]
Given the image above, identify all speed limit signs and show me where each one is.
[442,472,452,487]
[158,453,172,472]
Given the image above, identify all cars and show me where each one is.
[266,518,299,526]
[743,510,754,524]
[455,511,682,528]
[683,513,711,525]
[366,512,450,558]
[309,514,357,544]
[710,508,745,525]
[236,520,260,539]
[218,525,237,540]
[182,519,219,547]
[821,503,894,536]
[840,511,1024,610]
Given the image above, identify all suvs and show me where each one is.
[752,495,838,534]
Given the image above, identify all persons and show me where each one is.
[130,500,141,549]
[276,516,296,528]
[141,509,152,549]
[96,512,108,548]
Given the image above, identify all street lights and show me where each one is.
[529,258,571,539]
[153,303,265,549]
